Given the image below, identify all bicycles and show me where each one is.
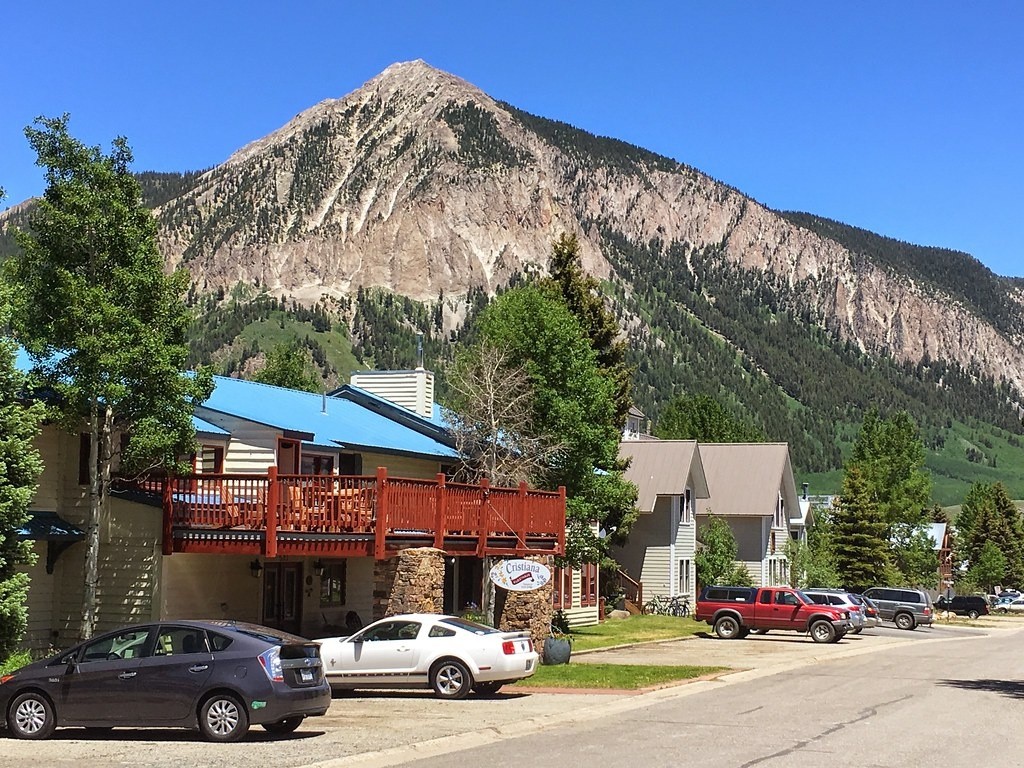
[642,590,691,619]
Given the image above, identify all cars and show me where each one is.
[0,619,332,743]
[986,589,1024,614]
[311,614,540,700]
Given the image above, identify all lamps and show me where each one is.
[250,558,262,578]
[313,557,325,576]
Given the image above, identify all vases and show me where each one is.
[543,638,571,665]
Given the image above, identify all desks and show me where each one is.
[307,491,355,533]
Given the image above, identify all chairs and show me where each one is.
[218,486,377,533]
[183,635,196,653]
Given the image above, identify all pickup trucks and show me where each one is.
[695,586,867,644]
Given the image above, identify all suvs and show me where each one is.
[932,594,989,619]
[782,587,865,638]
[862,587,935,630]
[848,592,882,635]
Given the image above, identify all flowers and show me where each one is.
[549,633,574,643]
